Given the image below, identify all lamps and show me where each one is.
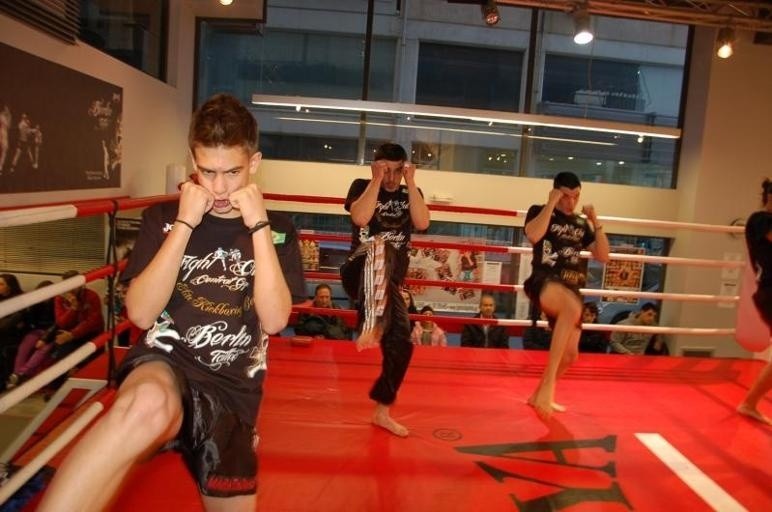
[570,5,594,45]
[480,0,502,25]
[716,28,735,60]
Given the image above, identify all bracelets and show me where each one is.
[594,225,603,231]
[160,218,194,234]
[244,220,272,234]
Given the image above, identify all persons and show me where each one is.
[108,245,133,346]
[611,301,661,356]
[296,282,352,341]
[523,302,609,356]
[460,294,509,349]
[402,289,448,347]
[32,91,294,512]
[0,103,43,175]
[339,142,431,438]
[522,171,610,421]
[735,176,772,428]
[0,269,102,403]
